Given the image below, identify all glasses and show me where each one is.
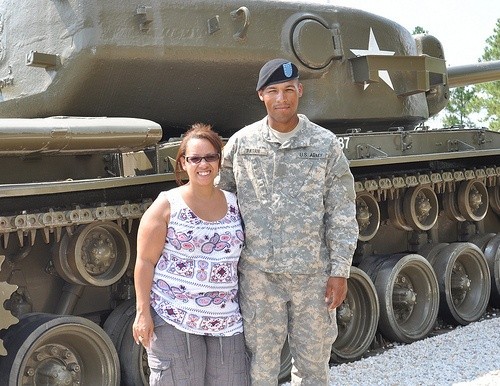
[183,153,220,164]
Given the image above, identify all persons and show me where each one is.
[132,123,248,386]
[215,59,358,386]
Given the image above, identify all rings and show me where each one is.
[138,335,144,342]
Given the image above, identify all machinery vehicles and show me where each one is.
[0,1,500,386]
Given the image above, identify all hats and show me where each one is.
[256,58,299,92]
